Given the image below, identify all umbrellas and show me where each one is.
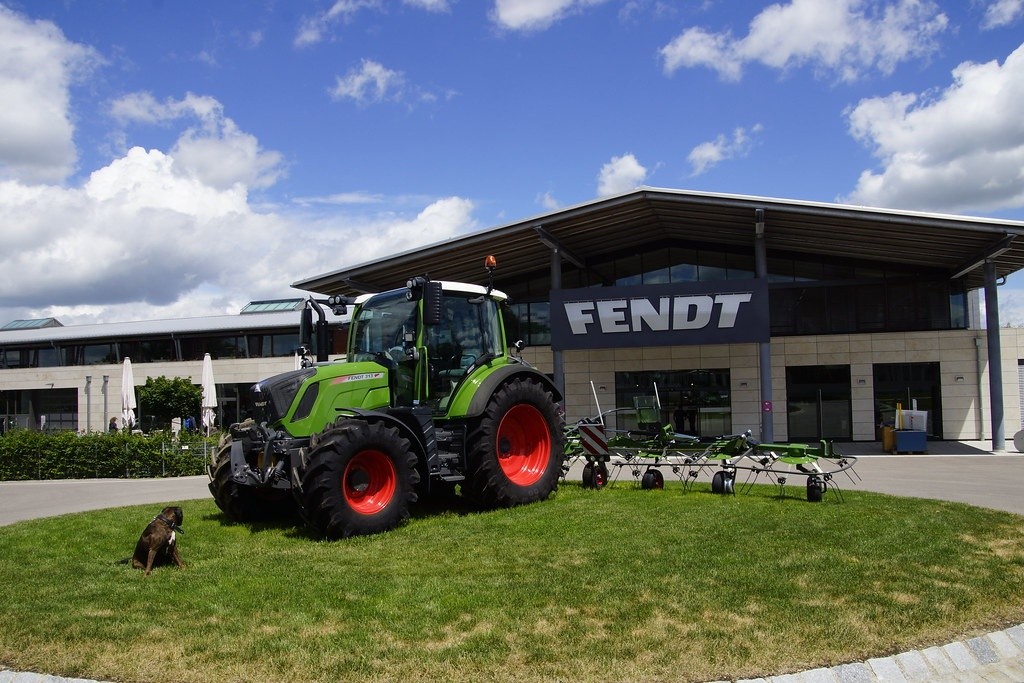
[295,346,306,371]
[121,356,137,430]
[201,353,218,437]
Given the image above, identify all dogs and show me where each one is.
[131,506,186,578]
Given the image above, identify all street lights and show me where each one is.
[86,375,91,436]
[103,375,109,433]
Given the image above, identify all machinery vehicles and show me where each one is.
[208,255,567,539]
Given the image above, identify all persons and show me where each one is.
[674,403,698,435]
[109,417,118,433]
[186,414,197,432]
